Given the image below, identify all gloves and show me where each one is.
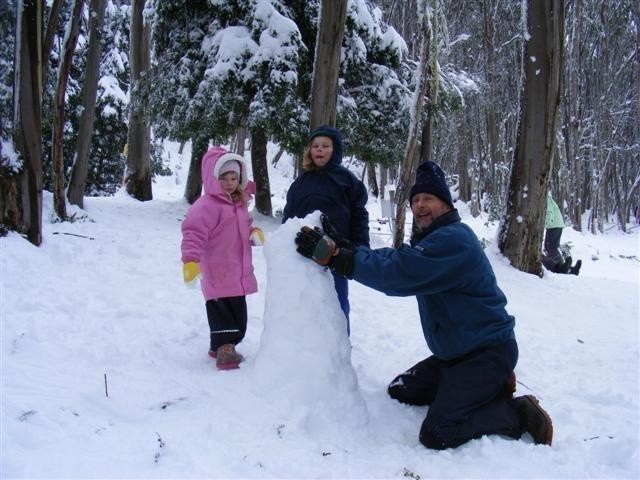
[183,261,203,282]
[248,228,264,246]
[295,226,356,280]
[321,214,354,249]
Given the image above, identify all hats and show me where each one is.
[219,160,241,182]
[409,161,453,210]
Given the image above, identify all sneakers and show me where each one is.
[502,371,516,399]
[208,349,244,362]
[215,344,240,370]
[515,395,553,446]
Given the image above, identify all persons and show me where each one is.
[541,255,582,275]
[281,123,372,349]
[295,160,554,450]
[180,146,268,373]
[543,190,565,266]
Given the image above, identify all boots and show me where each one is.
[569,260,581,275]
[556,257,572,273]
